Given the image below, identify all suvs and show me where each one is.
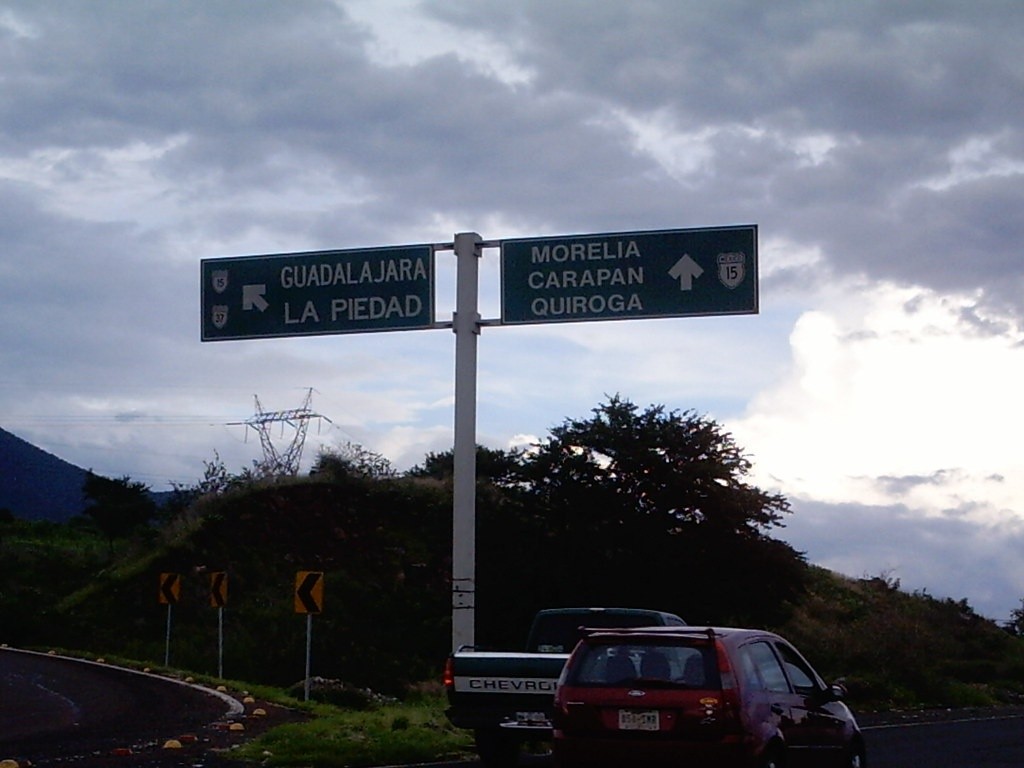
[553,626,867,768]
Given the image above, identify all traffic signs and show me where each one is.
[200,245,435,342]
[499,225,758,322]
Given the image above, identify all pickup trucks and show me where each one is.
[441,607,707,764]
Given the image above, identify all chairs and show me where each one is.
[605,656,637,683]
[640,652,671,680]
[683,654,705,685]
